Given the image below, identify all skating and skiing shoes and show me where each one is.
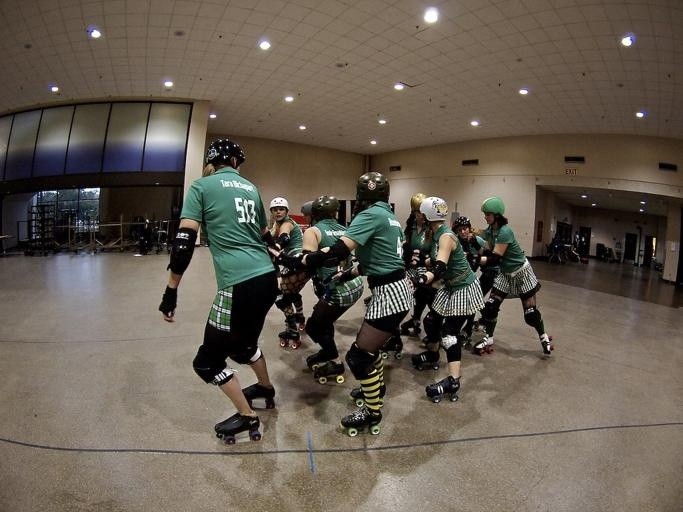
[306,349,339,372]
[380,337,404,360]
[412,351,441,371]
[471,335,495,356]
[241,383,275,410]
[425,375,462,404]
[540,331,556,358]
[313,360,346,385]
[278,328,302,350]
[400,317,421,336]
[348,385,386,409]
[215,413,261,445]
[341,407,383,437]
[294,314,305,332]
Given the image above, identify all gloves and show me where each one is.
[159,285,179,319]
[275,249,304,281]
[469,236,481,251]
[412,256,426,268]
[335,266,355,289]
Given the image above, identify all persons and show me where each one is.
[401,194,429,332]
[159,139,274,444]
[271,172,409,436]
[467,197,553,353]
[453,218,486,273]
[295,197,362,373]
[302,202,321,226]
[412,198,484,405]
[268,196,307,350]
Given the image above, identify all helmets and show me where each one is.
[300,201,316,216]
[203,139,247,166]
[420,197,449,221]
[480,196,504,217]
[311,195,338,213]
[454,217,473,228]
[268,197,289,212]
[358,172,388,203]
[410,193,426,214]
